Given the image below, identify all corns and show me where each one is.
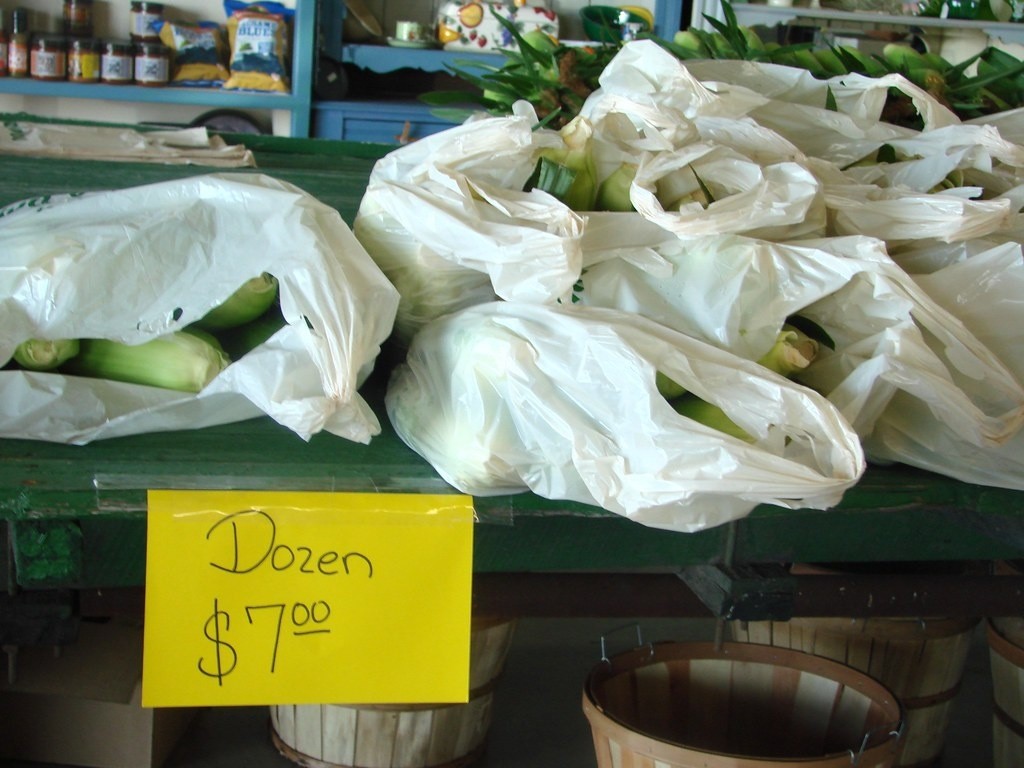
[12,268,288,395]
[474,25,1024,443]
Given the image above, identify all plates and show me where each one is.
[387,36,436,48]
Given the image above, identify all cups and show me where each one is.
[396,20,422,39]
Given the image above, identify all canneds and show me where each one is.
[0,0,172,87]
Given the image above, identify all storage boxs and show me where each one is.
[1,589,194,768]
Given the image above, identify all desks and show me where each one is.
[1,114,1024,580]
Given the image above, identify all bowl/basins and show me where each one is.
[344,0,384,44]
[580,5,651,43]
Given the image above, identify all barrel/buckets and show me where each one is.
[270,584,522,768]
[581,615,1024,768]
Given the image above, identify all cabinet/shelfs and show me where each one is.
[0,0,1024,144]
